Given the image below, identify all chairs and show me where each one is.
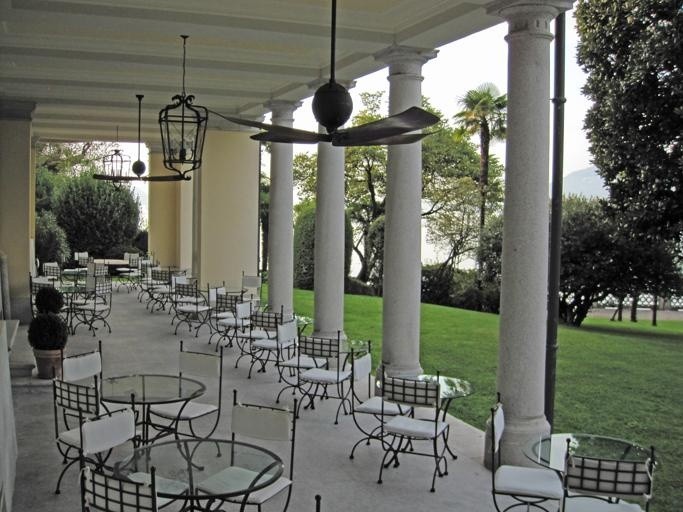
[27,252,283,337]
[490,391,566,510]
[563,437,658,511]
[52,327,477,511]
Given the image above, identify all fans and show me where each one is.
[209,1,445,147]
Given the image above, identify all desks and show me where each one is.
[522,433,658,512]
[255,312,313,361]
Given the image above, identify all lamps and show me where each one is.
[93,33,209,188]
